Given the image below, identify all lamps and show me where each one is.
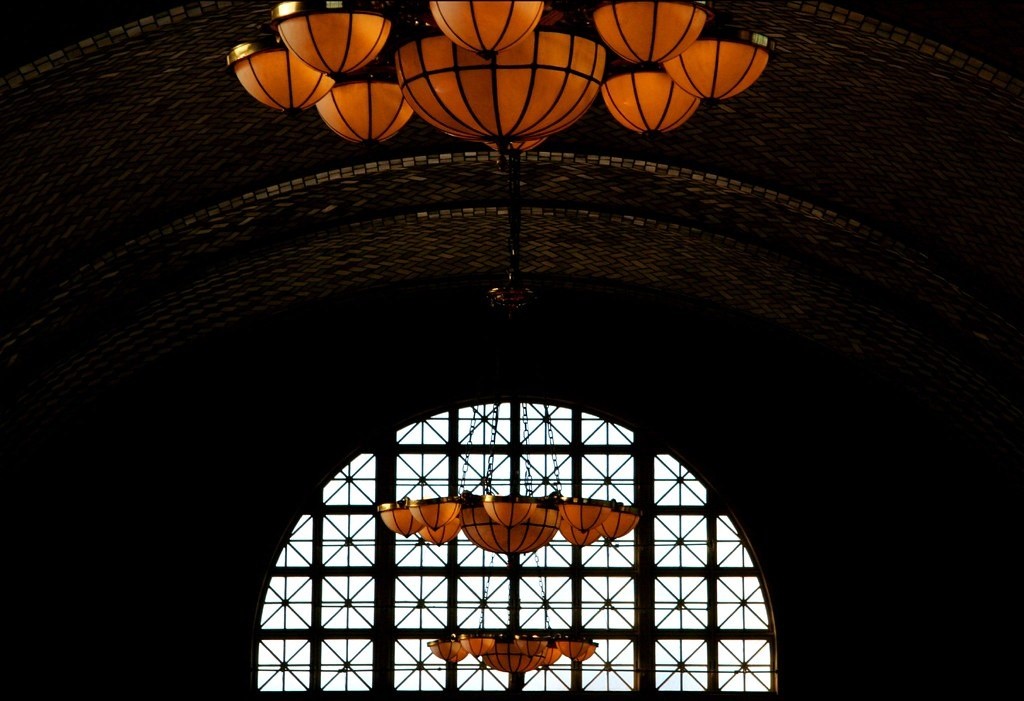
[376,399,643,554]
[427,554,598,672]
[227,0,778,328]
